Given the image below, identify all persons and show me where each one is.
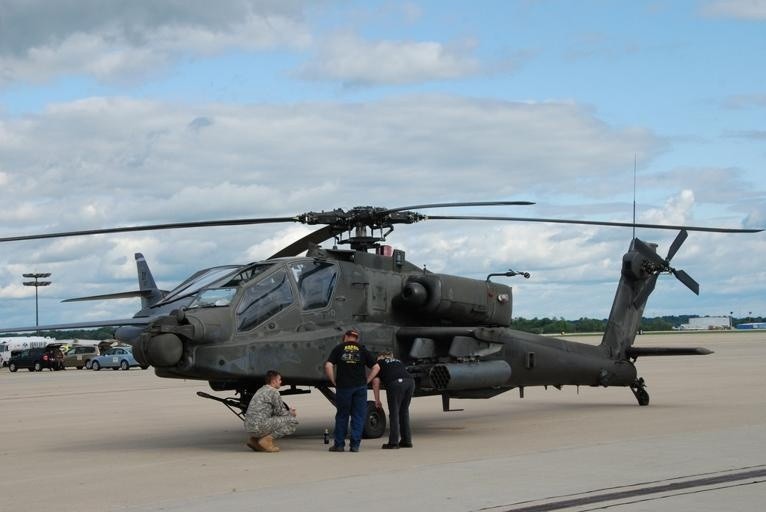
[48,349,58,371]
[55,350,66,370]
[322,329,381,454]
[241,369,300,454]
[371,349,416,450]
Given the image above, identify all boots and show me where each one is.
[258,436,280,452]
[246,436,263,452]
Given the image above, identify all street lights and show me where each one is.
[21,272,52,329]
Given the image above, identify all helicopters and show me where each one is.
[0,151,765,436]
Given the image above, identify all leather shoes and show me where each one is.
[382,443,399,450]
[349,445,359,452]
[328,444,345,452]
[399,441,413,448]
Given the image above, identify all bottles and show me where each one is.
[323,428,330,444]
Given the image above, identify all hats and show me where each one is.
[342,329,358,342]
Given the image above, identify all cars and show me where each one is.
[0,337,141,372]
